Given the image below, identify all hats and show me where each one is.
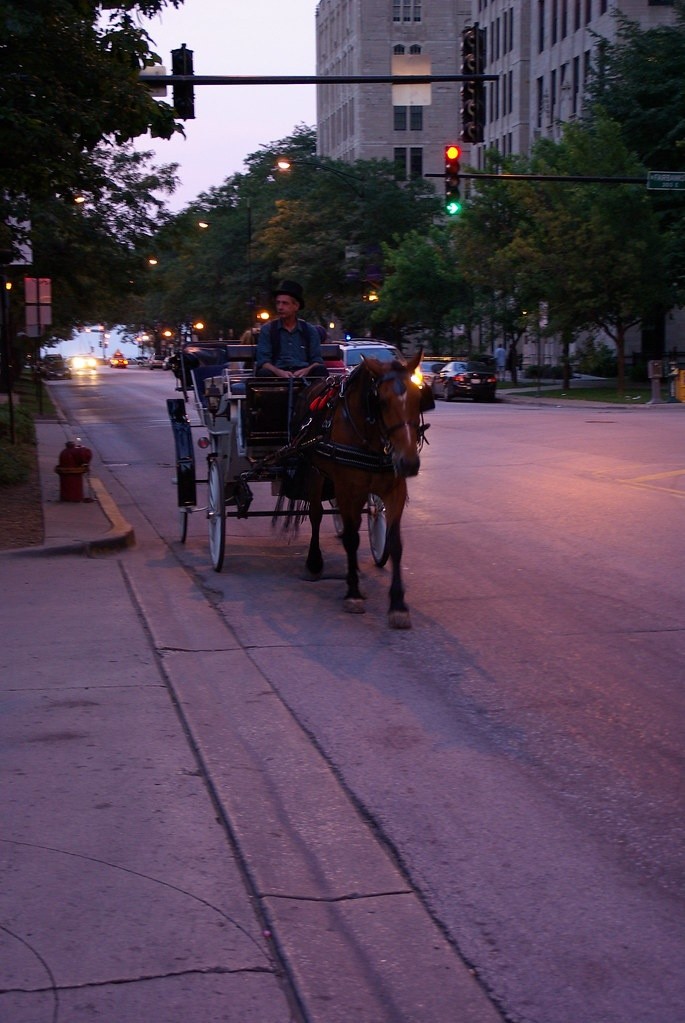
[272,280,306,310]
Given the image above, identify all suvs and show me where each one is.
[337,337,432,408]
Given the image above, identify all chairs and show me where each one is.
[243,357,338,435]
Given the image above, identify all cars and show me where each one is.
[431,359,498,403]
[40,353,72,380]
[111,357,129,368]
[134,352,176,370]
[407,359,447,392]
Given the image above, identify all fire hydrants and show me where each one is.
[54,442,94,502]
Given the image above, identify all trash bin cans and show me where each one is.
[667,357,685,403]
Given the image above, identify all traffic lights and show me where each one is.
[443,144,463,215]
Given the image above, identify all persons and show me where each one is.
[253,279,329,382]
[494,343,506,382]
[507,344,518,372]
[315,325,329,343]
[229,323,262,395]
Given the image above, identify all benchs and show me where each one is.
[217,344,340,419]
[186,346,344,422]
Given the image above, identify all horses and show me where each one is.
[283,346,423,630]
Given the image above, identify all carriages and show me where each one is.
[173,344,431,633]
